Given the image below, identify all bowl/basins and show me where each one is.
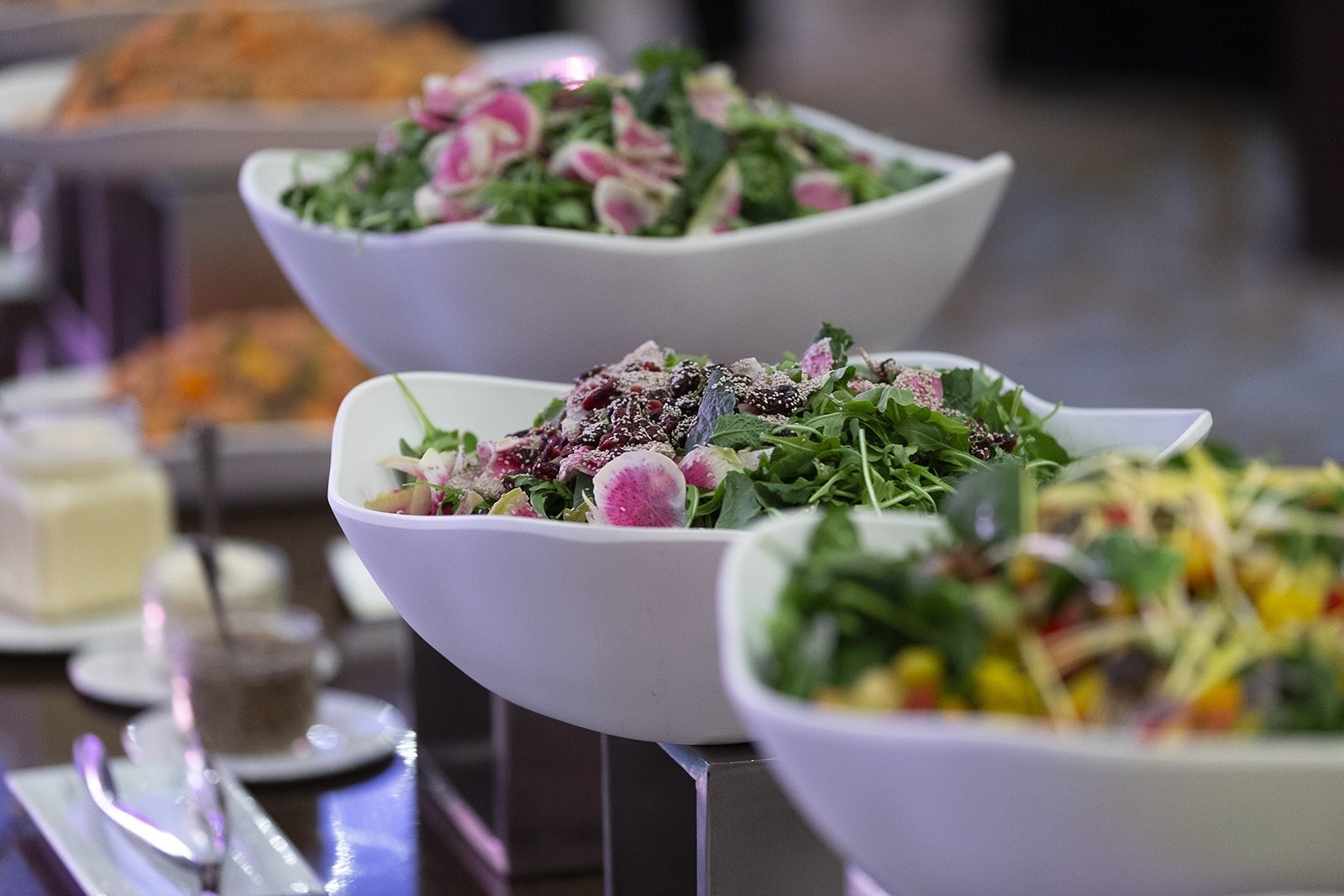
[712,510,1343,895]
[238,98,1015,388]
[327,354,1213,748]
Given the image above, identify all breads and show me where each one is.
[39,0,471,427]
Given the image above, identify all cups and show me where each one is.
[0,434,173,615]
[173,605,321,760]
[139,538,283,674]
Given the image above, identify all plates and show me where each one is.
[3,753,330,896]
[1,361,334,508]
[68,626,342,706]
[0,34,611,187]
[0,537,290,653]
[122,687,406,783]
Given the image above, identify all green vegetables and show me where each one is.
[385,323,1070,527]
[757,440,1344,734]
[279,38,945,236]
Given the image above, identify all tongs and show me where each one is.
[72,699,230,896]
